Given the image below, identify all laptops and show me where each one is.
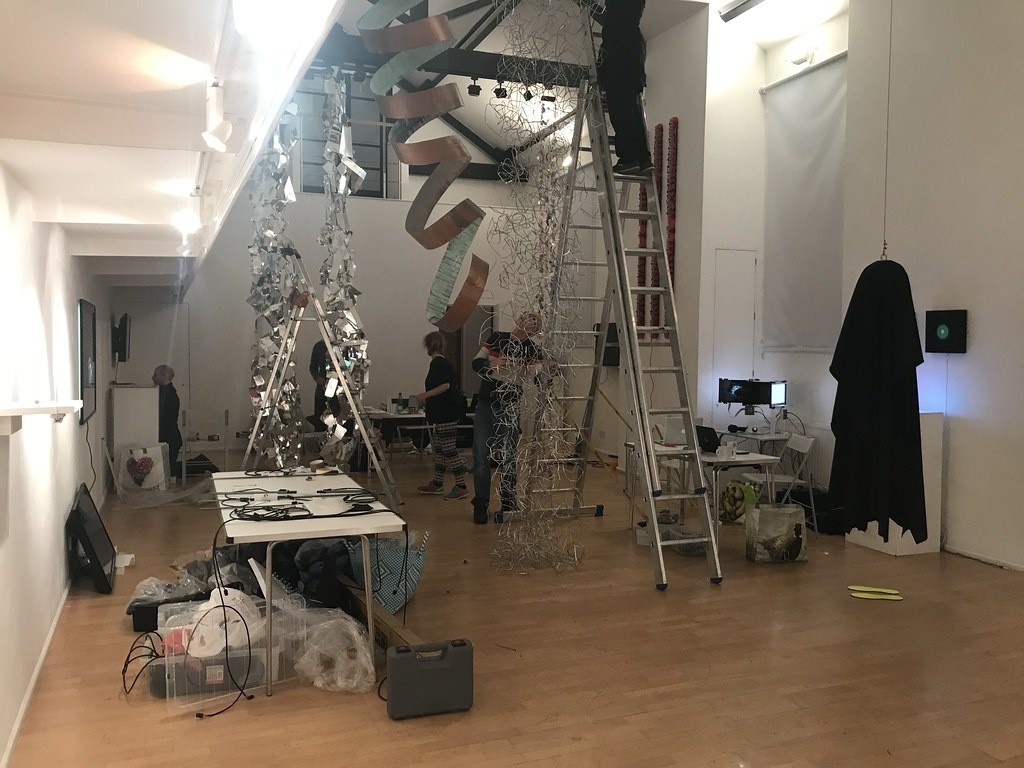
[654,417,703,445]
[696,425,749,454]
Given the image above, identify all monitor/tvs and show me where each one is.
[77,299,96,425]
[117,313,130,362]
[71,482,116,594]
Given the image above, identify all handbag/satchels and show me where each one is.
[342,530,429,616]
[746,502,808,564]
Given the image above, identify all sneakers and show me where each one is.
[417,481,444,494]
[444,486,470,500]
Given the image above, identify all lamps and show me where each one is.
[203,118,236,154]
[523,82,533,101]
[467,76,482,96]
[542,84,557,103]
[494,78,506,97]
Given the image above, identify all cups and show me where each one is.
[717,446,732,461]
[727,441,737,460]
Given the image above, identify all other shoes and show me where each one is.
[196,454,219,472]
[613,161,641,175]
[474,507,488,523]
[637,160,655,176]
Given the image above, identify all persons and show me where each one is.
[152,365,219,475]
[471,313,559,524]
[418,332,470,500]
[309,339,339,432]
[575,0,656,176]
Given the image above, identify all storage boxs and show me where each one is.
[121,581,289,702]
[338,571,432,665]
[633,512,711,555]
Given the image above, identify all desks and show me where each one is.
[715,428,809,503]
[208,460,407,688]
[678,452,780,556]
[360,409,473,464]
[623,437,701,505]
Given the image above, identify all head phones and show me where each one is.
[728,424,747,433]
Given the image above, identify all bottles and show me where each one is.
[396,392,403,412]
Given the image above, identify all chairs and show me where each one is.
[741,432,819,537]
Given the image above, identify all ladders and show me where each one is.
[494,0,723,591]
[239,247,405,515]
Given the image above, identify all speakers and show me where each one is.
[595,323,619,366]
